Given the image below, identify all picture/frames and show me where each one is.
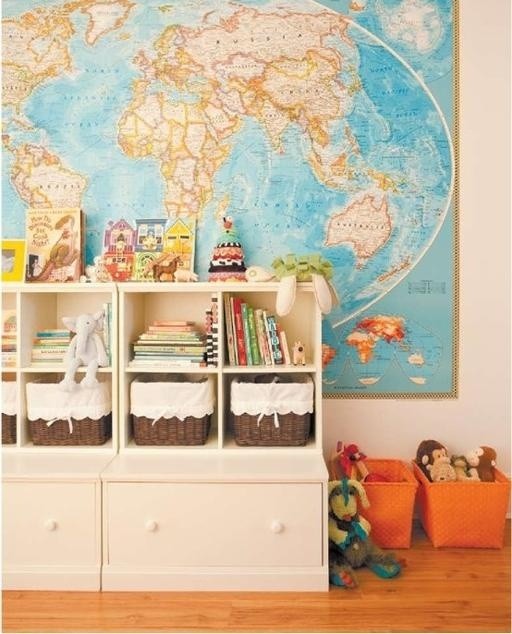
[1,239,26,284]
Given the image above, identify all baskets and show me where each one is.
[129,374,216,446]
[229,376,314,446]
[26,378,110,446]
[2,381,16,443]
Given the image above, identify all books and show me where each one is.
[26,208,87,281]
[2,290,291,366]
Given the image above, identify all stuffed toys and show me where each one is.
[417,438,456,484]
[468,445,501,483]
[245,252,340,317]
[56,310,110,392]
[451,454,472,481]
[292,340,308,366]
[328,477,400,590]
[174,270,199,281]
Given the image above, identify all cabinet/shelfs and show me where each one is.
[101,281,329,592]
[2,283,119,590]
